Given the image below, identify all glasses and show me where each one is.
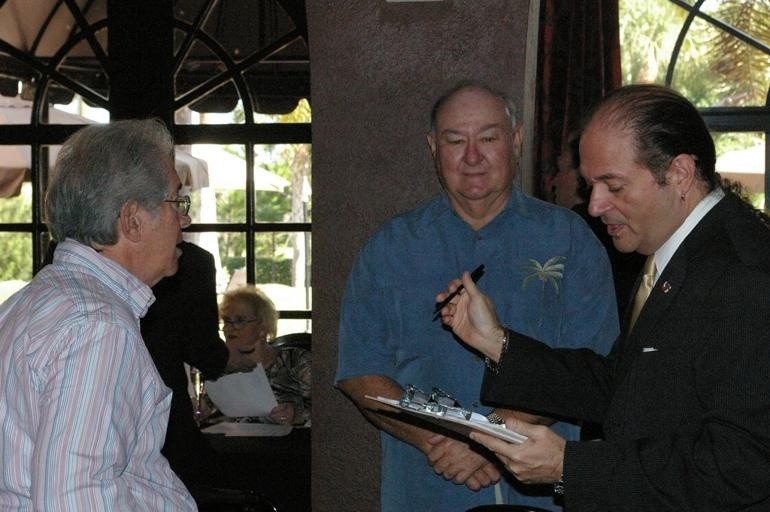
[164,196,190,217]
[219,317,262,331]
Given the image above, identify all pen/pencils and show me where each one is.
[432,264,486,316]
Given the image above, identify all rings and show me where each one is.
[280,421,289,425]
[279,416,288,420]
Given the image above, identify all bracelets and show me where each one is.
[485,326,507,375]
[551,471,564,496]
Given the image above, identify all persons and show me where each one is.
[434,82,770,512]
[40,225,257,501]
[331,80,621,509]
[191,285,312,427]
[0,115,200,512]
